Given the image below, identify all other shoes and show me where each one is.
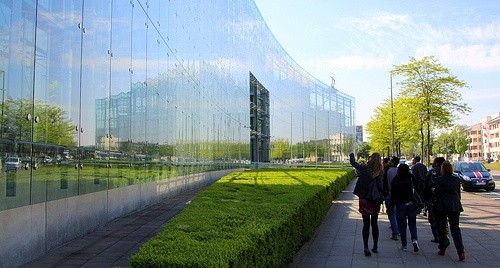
[412,240,419,253]
[423,212,427,216]
[446,235,450,246]
[401,246,407,252]
[390,234,399,240]
[372,248,377,253]
[364,248,371,256]
[431,239,438,243]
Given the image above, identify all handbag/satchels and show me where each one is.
[410,177,424,210]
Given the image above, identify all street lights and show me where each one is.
[390,65,422,157]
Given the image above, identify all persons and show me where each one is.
[349,152,465,262]
[488,157,491,163]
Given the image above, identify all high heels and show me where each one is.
[438,249,446,256]
[459,253,464,261]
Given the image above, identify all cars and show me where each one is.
[453,161,495,192]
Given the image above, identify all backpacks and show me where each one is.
[367,174,385,204]
[414,173,434,202]
